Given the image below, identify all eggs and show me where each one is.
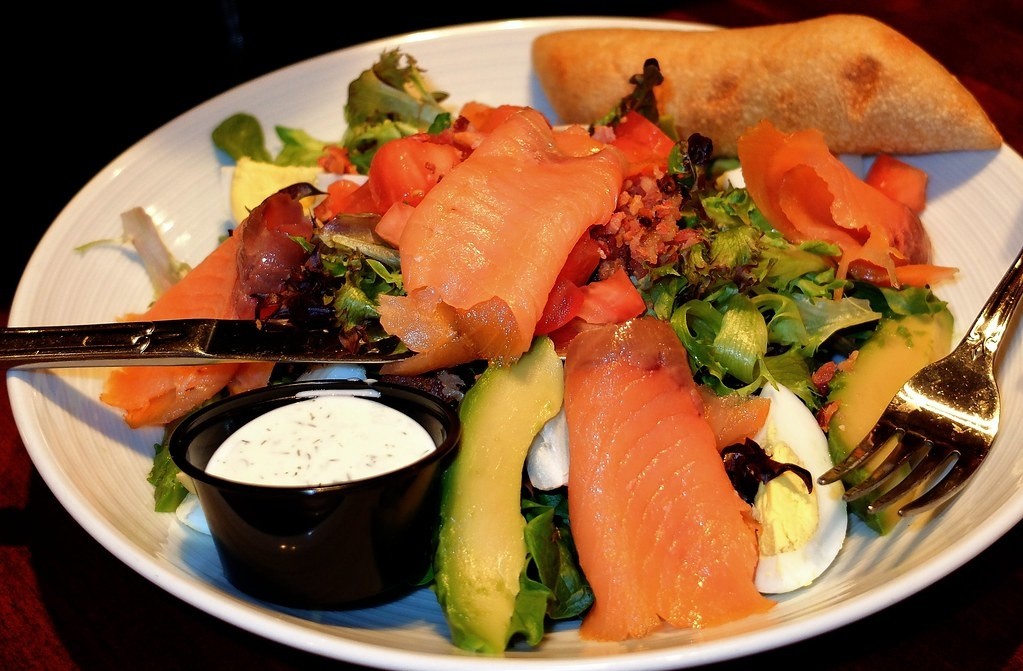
[746,377,850,593]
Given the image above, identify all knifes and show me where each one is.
[0,318,417,365]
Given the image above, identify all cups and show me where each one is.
[168,380,462,616]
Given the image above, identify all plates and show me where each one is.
[3,15,1023,670]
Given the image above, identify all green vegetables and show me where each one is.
[78,48,954,657]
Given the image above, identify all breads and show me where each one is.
[532,17,1005,156]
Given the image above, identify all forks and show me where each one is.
[819,248,1023,518]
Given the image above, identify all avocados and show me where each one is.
[431,333,564,655]
[825,306,954,533]
